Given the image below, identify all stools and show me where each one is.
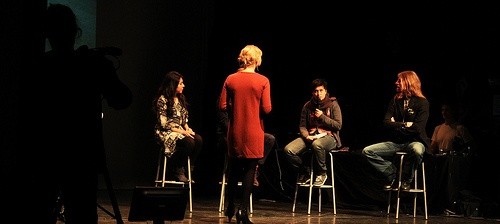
[292,149,337,215]
[153,137,193,213]
[218,152,254,214]
[386,152,428,220]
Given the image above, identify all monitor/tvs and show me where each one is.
[128,186,190,224]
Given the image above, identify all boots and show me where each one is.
[186,165,195,184]
[174,167,190,182]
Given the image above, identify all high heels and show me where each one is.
[225,205,235,223]
[236,207,254,224]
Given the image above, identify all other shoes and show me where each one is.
[296,174,310,185]
[313,171,328,187]
[401,178,411,191]
[384,178,397,191]
[253,173,260,186]
[443,208,465,219]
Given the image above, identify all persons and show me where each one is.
[152,72,202,185]
[45,4,133,224]
[426,104,474,214]
[361,71,430,194]
[284,79,342,187]
[218,44,275,224]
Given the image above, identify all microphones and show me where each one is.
[318,102,322,109]
